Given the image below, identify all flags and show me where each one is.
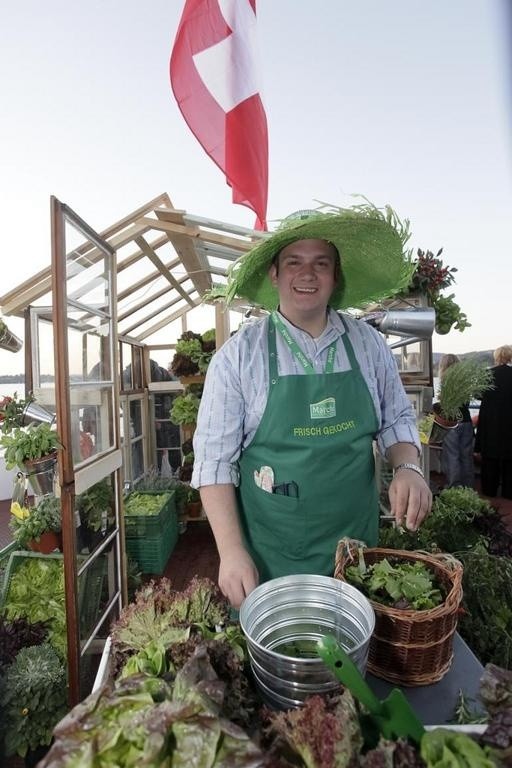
[169,0,271,231]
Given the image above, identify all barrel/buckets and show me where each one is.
[21,451,59,497]
[236,575,377,716]
[20,401,56,429]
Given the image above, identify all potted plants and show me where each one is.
[0,389,200,577]
[431,357,496,445]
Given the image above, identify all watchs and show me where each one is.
[392,463,425,478]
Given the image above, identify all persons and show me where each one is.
[83,344,182,474]
[439,346,512,499]
[189,204,433,613]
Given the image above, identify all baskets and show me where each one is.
[1,546,101,645]
[119,488,180,577]
[328,537,463,688]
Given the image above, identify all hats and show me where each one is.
[203,195,417,315]
